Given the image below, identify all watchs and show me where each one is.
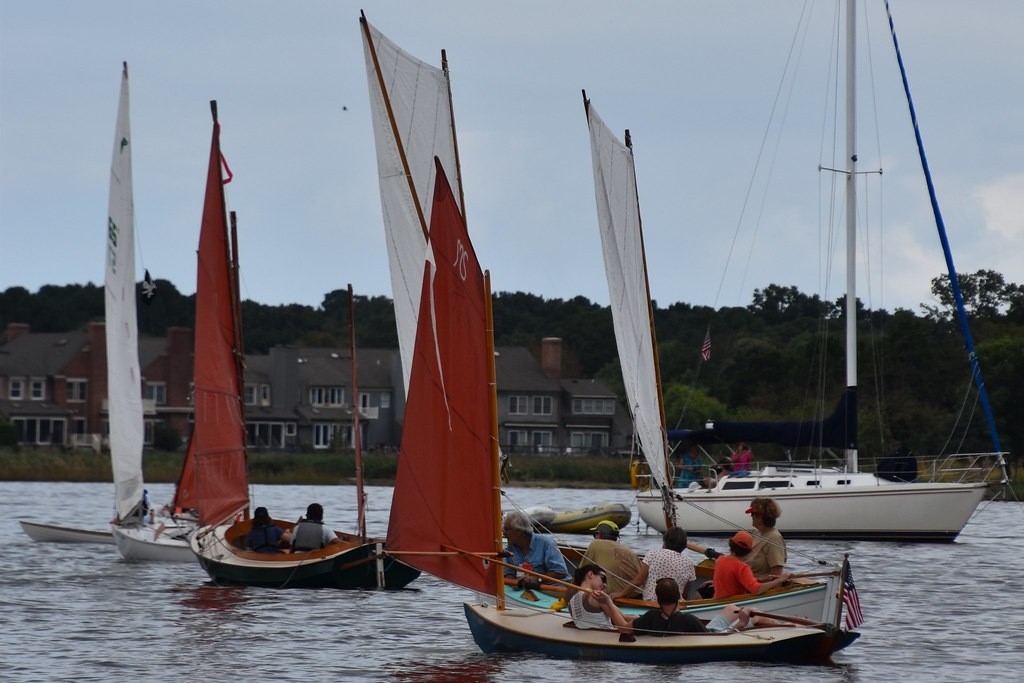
[537,577,543,583]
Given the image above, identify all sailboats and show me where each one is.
[354,9,864,664]
[18,60,195,545]
[635,0,1013,543]
[104,100,420,589]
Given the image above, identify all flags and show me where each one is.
[836,560,865,633]
[702,331,711,361]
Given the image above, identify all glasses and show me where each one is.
[503,528,513,535]
[592,530,600,538]
[594,573,607,584]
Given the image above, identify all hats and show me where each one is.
[252,507,272,525]
[745,502,762,514]
[732,531,752,550]
[590,520,619,535]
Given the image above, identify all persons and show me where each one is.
[712,531,791,597]
[742,498,788,581]
[243,507,292,554]
[554,565,626,629]
[731,442,753,471]
[608,526,697,603]
[289,503,347,552]
[592,577,808,636]
[502,511,573,598]
[131,489,148,516]
[578,520,643,598]
[703,457,732,489]
[677,447,704,488]
